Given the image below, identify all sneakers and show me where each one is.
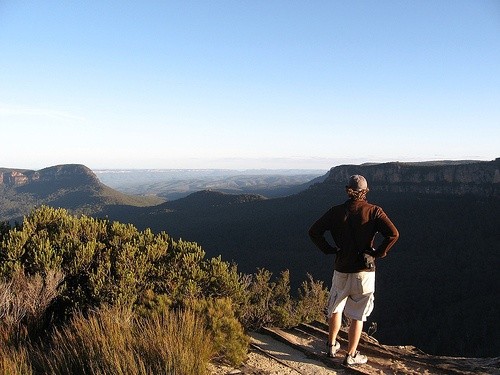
[344,352,368,365]
[326,340,340,358]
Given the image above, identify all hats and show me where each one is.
[348,175,367,189]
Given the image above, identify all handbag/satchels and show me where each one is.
[362,249,376,264]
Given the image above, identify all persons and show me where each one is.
[309,174,400,365]
